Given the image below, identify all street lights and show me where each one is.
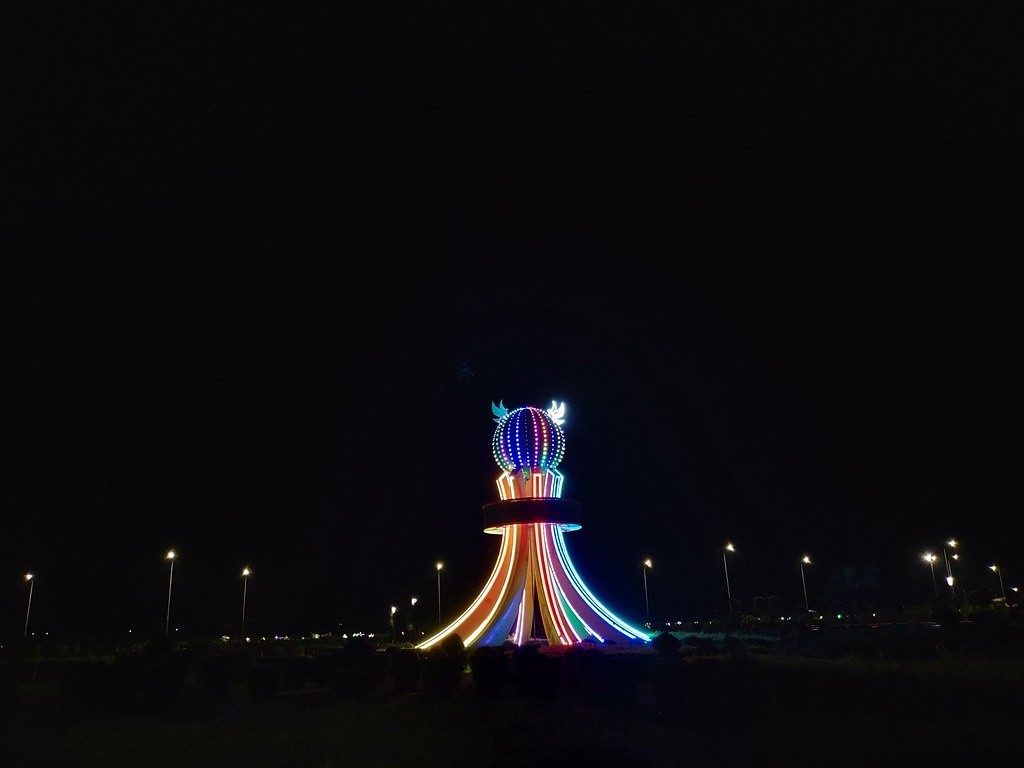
[801,556,809,612]
[241,568,249,637]
[436,562,443,625]
[723,544,735,612]
[644,559,651,619]
[944,540,957,593]
[165,550,175,634]
[24,573,34,636]
[929,554,939,598]
[991,564,1005,601]
